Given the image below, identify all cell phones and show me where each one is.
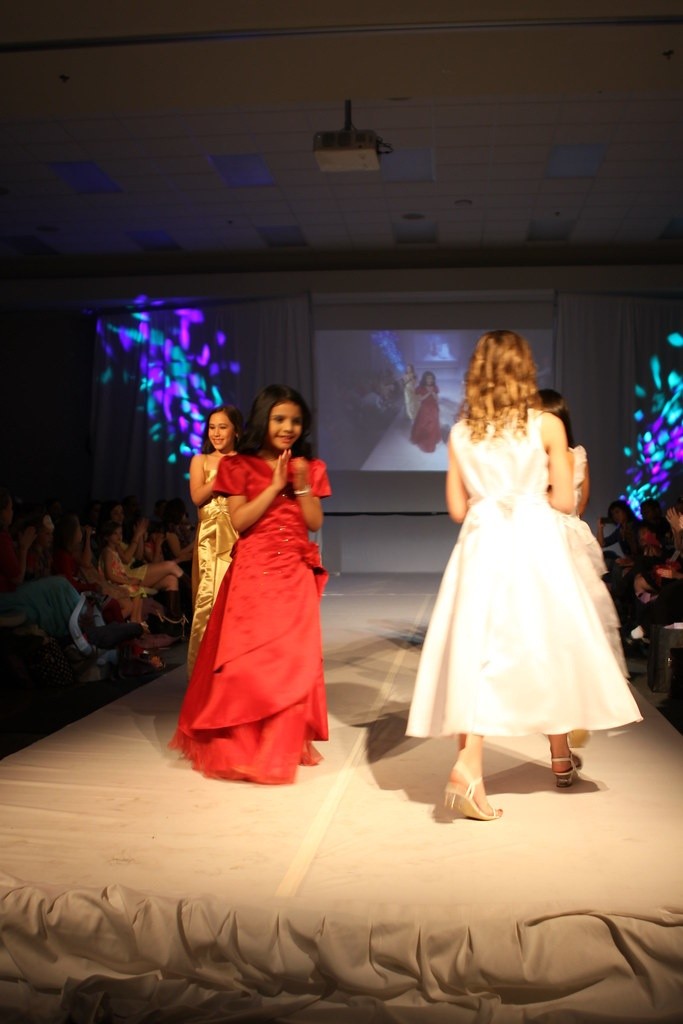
[601,517,615,524]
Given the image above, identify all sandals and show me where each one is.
[441,763,505,822]
[549,750,583,787]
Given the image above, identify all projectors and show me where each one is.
[314,130,381,173]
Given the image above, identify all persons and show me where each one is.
[170,383,332,785]
[534,386,628,753]
[0,484,200,692]
[185,401,248,683]
[594,456,683,682]
[336,362,442,454]
[404,329,645,822]
